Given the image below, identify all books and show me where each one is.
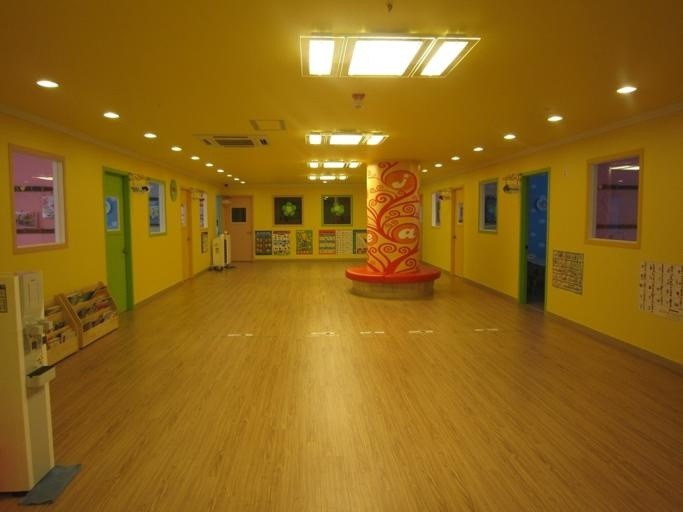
[30,283,119,353]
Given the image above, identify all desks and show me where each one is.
[527,256,545,296]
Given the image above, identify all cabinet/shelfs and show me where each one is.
[62,280,119,348]
[44,295,79,367]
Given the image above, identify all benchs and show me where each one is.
[346,265,441,298]
